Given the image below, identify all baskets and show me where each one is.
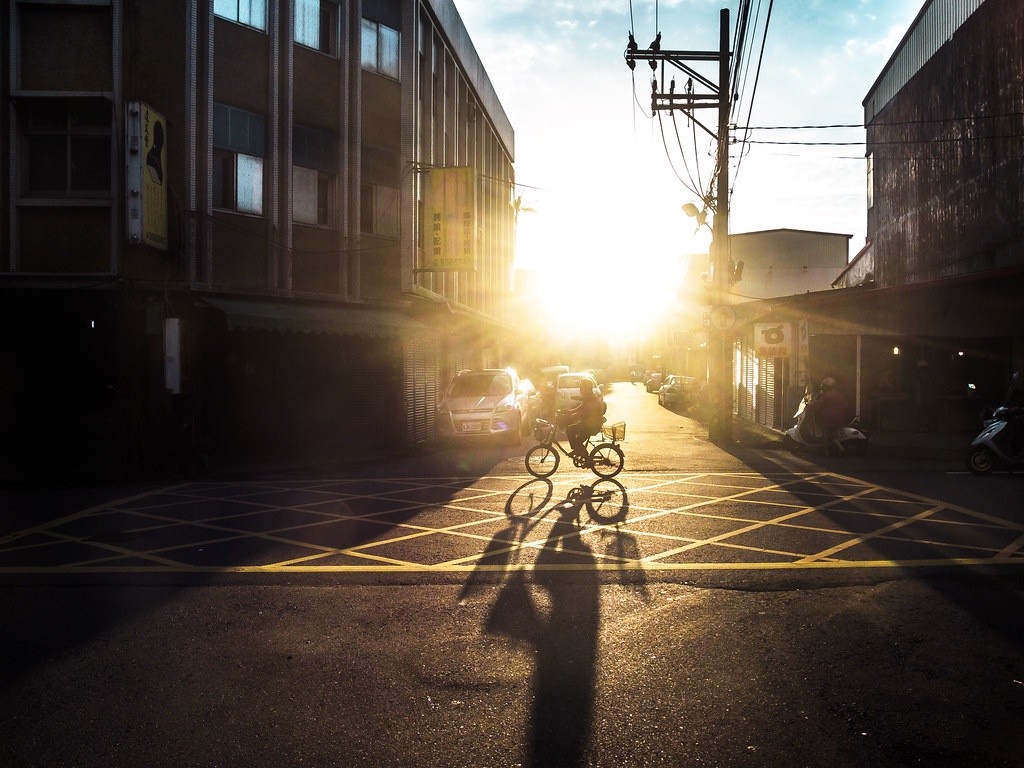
[534,419,552,442]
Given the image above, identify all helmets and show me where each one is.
[821,377,836,395]
[1010,371,1024,393]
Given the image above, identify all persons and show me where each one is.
[631,369,635,375]
[557,378,602,468]
[812,377,849,429]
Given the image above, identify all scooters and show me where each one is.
[964,405,1022,476]
[784,392,865,458]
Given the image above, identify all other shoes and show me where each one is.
[566,451,575,456]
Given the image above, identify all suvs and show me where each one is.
[435,367,530,447]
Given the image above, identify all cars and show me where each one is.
[643,371,696,407]
[520,366,606,421]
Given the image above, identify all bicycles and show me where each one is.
[525,408,626,478]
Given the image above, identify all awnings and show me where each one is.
[202,294,428,338]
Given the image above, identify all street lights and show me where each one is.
[682,202,723,441]
